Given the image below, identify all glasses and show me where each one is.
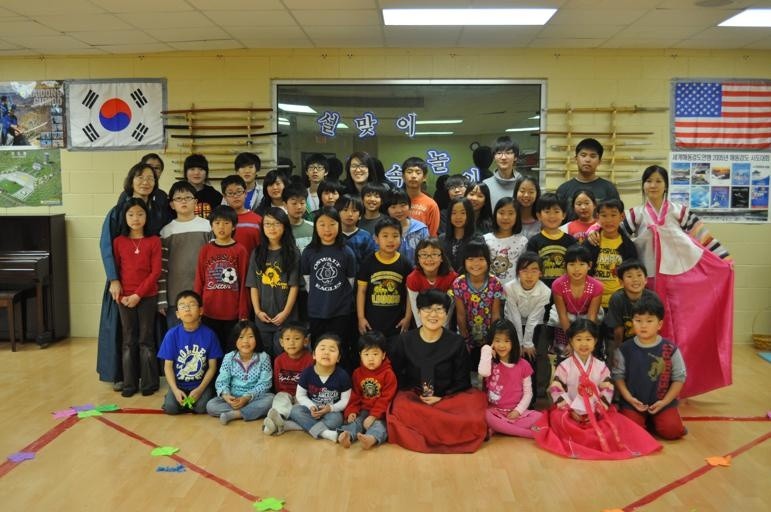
[171,196,195,202]
[308,165,325,171]
[263,221,281,227]
[422,306,445,314]
[225,190,245,197]
[417,254,442,259]
[494,151,514,159]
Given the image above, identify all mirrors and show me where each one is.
[271,79,545,198]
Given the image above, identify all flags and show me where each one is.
[674,83,771,149]
[68,83,164,147]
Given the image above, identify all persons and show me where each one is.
[0,94,12,122]
[481,136,735,462]
[96,152,504,455]
[1,105,23,145]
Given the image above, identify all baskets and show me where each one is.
[752,307,771,350]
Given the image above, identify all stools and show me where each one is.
[0,290,25,351]
